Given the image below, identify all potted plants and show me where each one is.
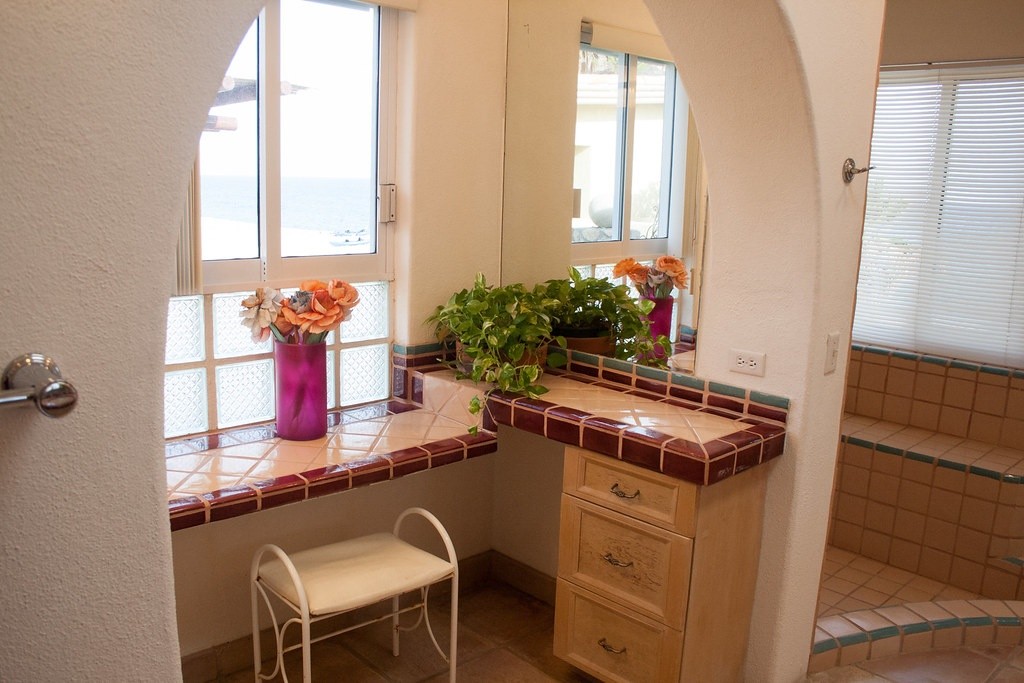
[421,272,562,439]
[533,266,680,372]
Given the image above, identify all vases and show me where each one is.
[274,339,327,441]
[635,295,674,364]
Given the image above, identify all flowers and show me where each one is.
[612,255,690,299]
[237,277,361,344]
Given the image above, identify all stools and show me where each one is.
[249,506,458,683]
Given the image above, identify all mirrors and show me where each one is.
[499,0,708,375]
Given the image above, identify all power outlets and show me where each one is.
[824,331,840,374]
[729,348,767,377]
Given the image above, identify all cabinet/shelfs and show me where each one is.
[553,444,698,683]
